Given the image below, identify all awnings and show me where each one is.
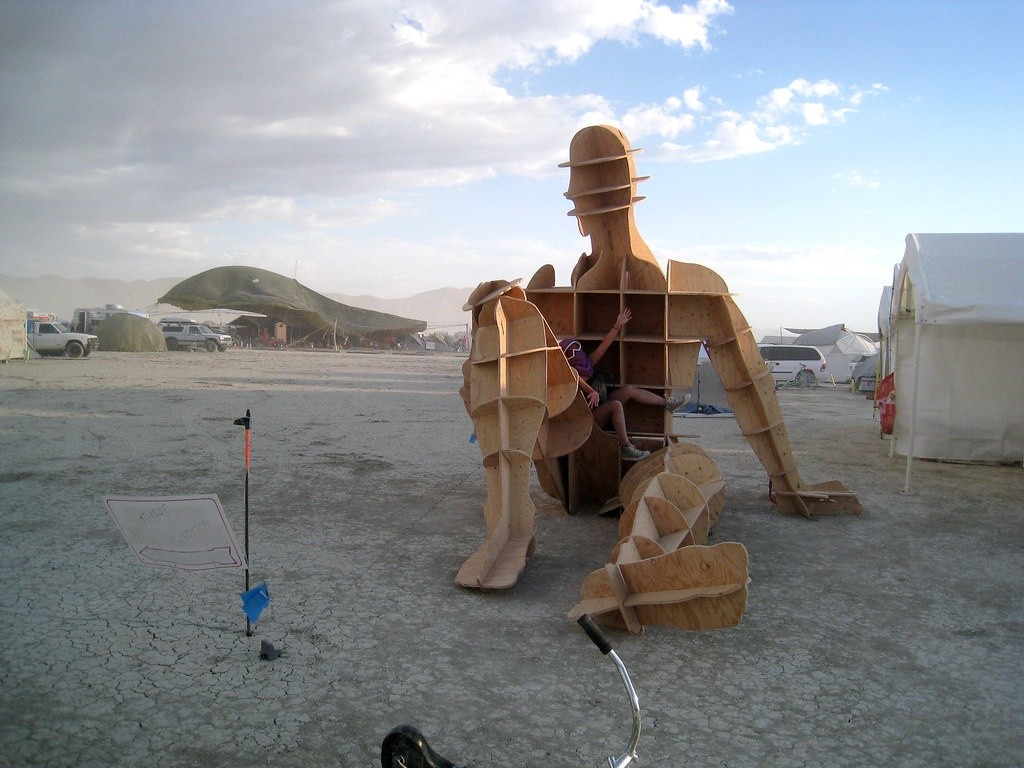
[153,264,431,346]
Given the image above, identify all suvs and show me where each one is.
[160,317,232,353]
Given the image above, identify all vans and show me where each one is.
[757,343,827,388]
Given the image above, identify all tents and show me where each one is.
[785,326,877,383]
[876,231,1024,499]
[90,312,167,350]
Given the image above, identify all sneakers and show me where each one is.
[662,393,691,414]
[621,442,650,462]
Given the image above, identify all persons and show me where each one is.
[559,305,691,462]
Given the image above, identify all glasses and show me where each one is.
[564,341,581,360]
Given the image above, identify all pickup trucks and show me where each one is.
[26,322,99,358]
